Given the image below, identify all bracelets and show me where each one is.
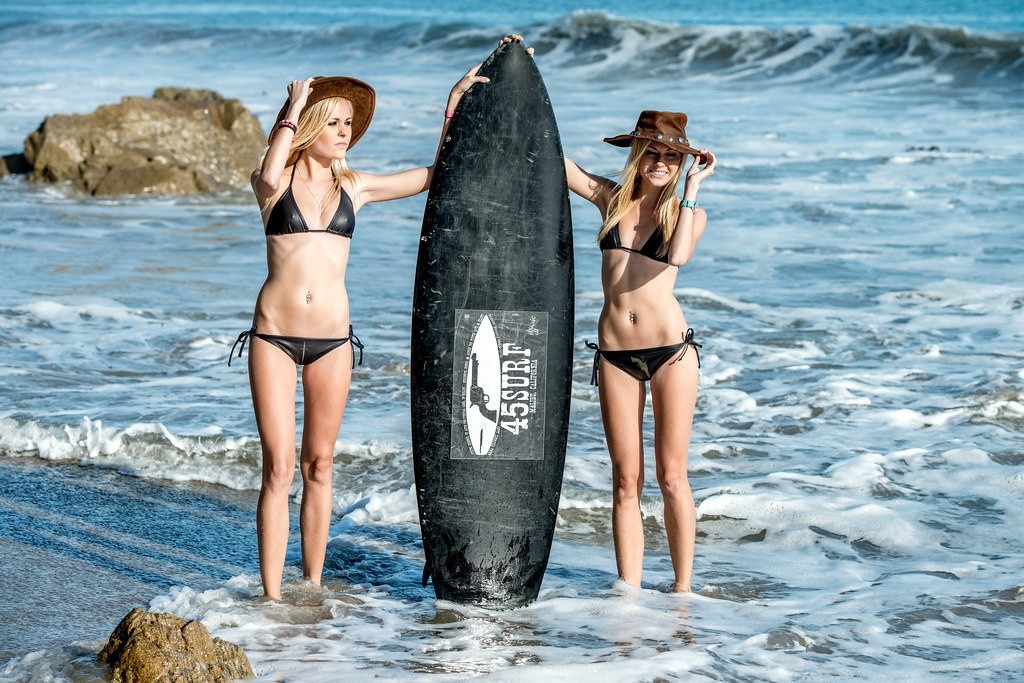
[445,110,455,118]
[279,119,298,135]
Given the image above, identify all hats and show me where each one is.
[268,76,375,152]
[603,111,708,166]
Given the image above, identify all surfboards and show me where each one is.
[408,37,577,613]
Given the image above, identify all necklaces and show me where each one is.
[295,162,336,210]
[634,204,652,229]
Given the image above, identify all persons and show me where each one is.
[498,34,717,593]
[248,64,491,601]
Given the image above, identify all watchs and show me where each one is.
[679,200,699,214]
